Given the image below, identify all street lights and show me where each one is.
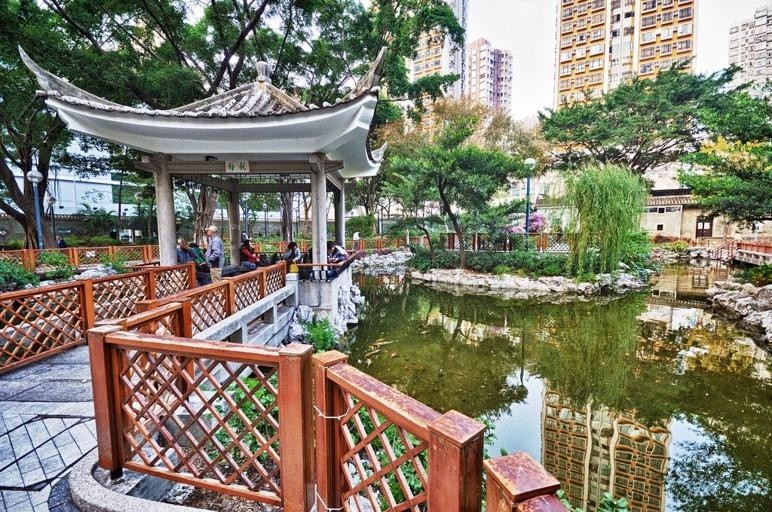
[524,157,536,249]
[379,197,386,237]
[134,191,142,225]
[49,196,57,239]
[262,202,268,235]
[26,156,43,248]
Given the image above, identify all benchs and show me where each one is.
[197,263,245,285]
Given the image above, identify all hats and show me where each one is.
[204,225,218,232]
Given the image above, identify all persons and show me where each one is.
[56,235,61,248]
[60,236,67,248]
[733,229,744,250]
[174,224,350,287]
[352,230,361,250]
